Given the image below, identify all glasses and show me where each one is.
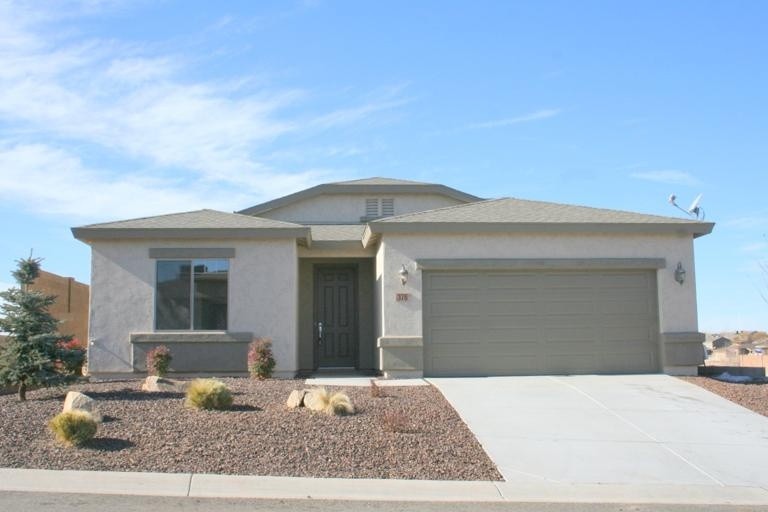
[675,262,686,286]
[398,264,409,287]
